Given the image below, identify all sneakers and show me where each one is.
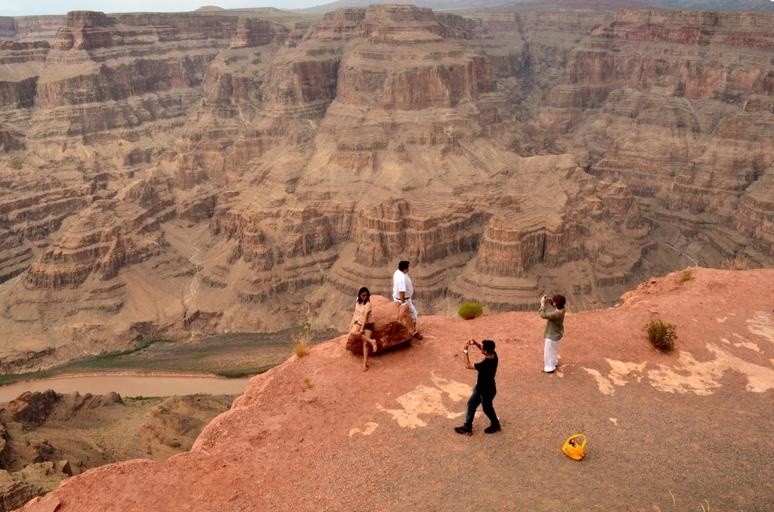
[485,423,501,433]
[455,426,472,435]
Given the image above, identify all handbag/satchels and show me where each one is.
[561,434,587,460]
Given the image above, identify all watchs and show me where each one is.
[463,349,468,354]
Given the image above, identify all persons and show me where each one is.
[455,337,501,436]
[538,294,566,373]
[392,261,423,339]
[352,287,377,372]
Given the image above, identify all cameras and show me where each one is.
[466,339,471,345]
